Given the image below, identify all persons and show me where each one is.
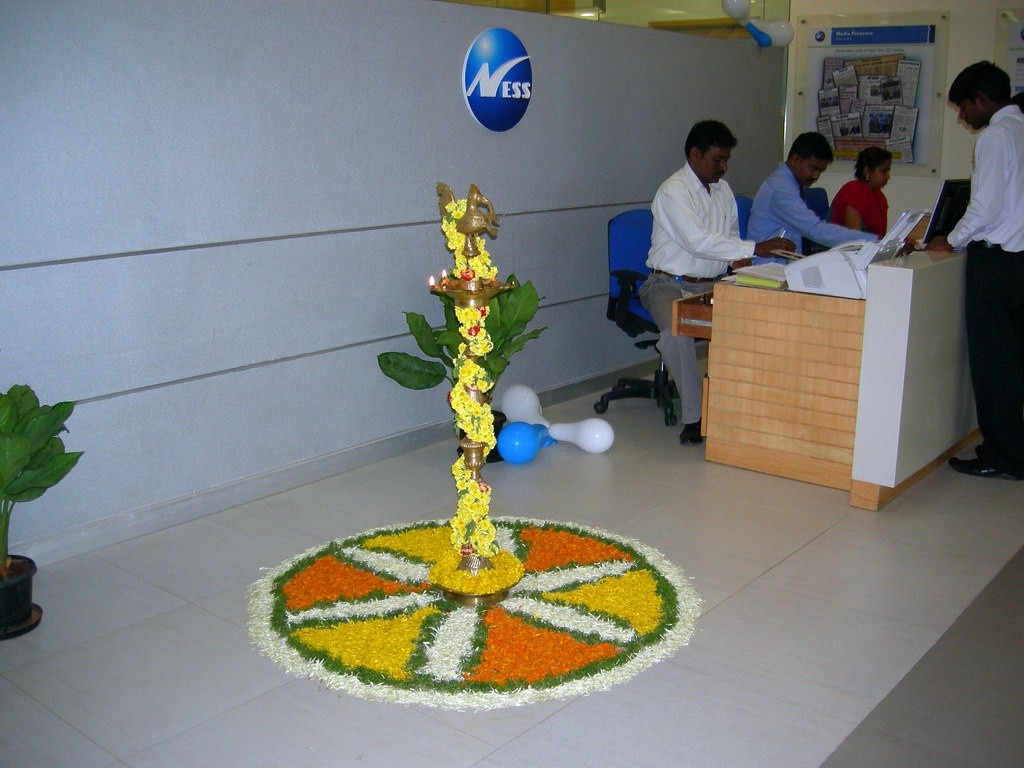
[920,59,1024,482]
[743,131,892,266]
[637,119,797,446]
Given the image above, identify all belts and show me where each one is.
[651,268,727,284]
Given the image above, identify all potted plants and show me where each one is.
[0,384,86,639]
[377,273,548,462]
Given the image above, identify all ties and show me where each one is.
[799,187,807,255]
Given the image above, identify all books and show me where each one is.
[732,262,792,290]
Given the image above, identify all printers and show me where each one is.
[783,238,909,300]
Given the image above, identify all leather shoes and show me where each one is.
[680,421,702,445]
[975,443,984,458]
[948,456,1024,480]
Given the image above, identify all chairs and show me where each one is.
[799,187,828,256]
[735,194,755,242]
[593,209,682,426]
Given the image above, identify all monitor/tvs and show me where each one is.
[923,179,973,244]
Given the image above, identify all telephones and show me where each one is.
[827,238,867,252]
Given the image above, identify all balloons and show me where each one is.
[494,384,615,464]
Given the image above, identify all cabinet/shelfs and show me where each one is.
[671,281,865,490]
